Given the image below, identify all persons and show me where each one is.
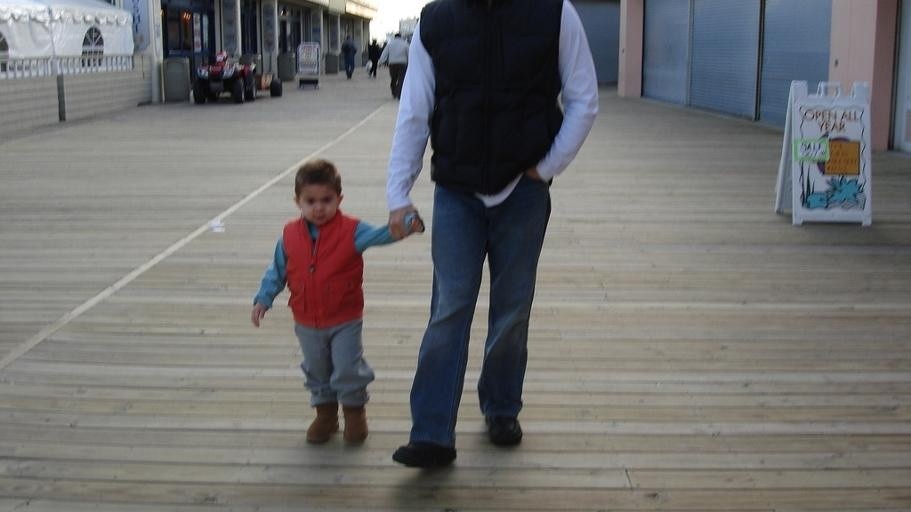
[342,34,357,79]
[386,0,600,467]
[367,32,412,100]
[250,160,425,444]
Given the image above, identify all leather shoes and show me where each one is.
[393,441,456,467]
[485,413,522,445]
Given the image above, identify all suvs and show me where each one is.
[193,49,258,103]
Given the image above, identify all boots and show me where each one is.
[306,401,338,442]
[342,405,368,442]
